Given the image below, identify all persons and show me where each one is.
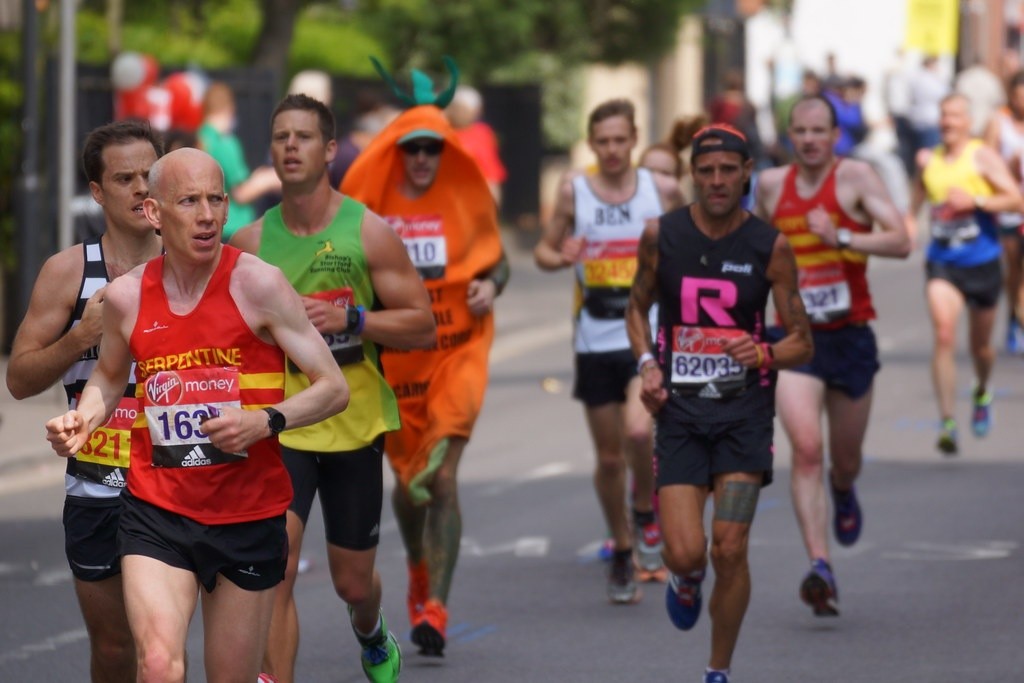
[737,0,912,258]
[752,95,912,617]
[338,104,511,660]
[6,115,353,682]
[223,93,435,683]
[533,67,761,606]
[165,70,506,247]
[626,122,815,683]
[905,93,1024,454]
[889,44,1024,359]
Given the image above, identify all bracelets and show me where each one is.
[755,344,765,368]
[636,353,654,375]
[974,194,985,214]
[352,305,366,336]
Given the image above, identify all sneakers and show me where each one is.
[405,557,430,616]
[800,559,840,617]
[347,604,402,683]
[631,506,666,582]
[664,532,710,631]
[827,467,863,546]
[409,597,447,654]
[937,417,957,453]
[1007,310,1024,356]
[606,544,636,603]
[971,380,991,435]
[703,664,730,683]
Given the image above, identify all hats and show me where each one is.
[690,121,752,197]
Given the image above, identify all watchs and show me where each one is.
[346,303,360,334]
[834,228,852,253]
[261,407,286,438]
[760,342,775,369]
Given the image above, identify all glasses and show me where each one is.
[401,141,441,156]
